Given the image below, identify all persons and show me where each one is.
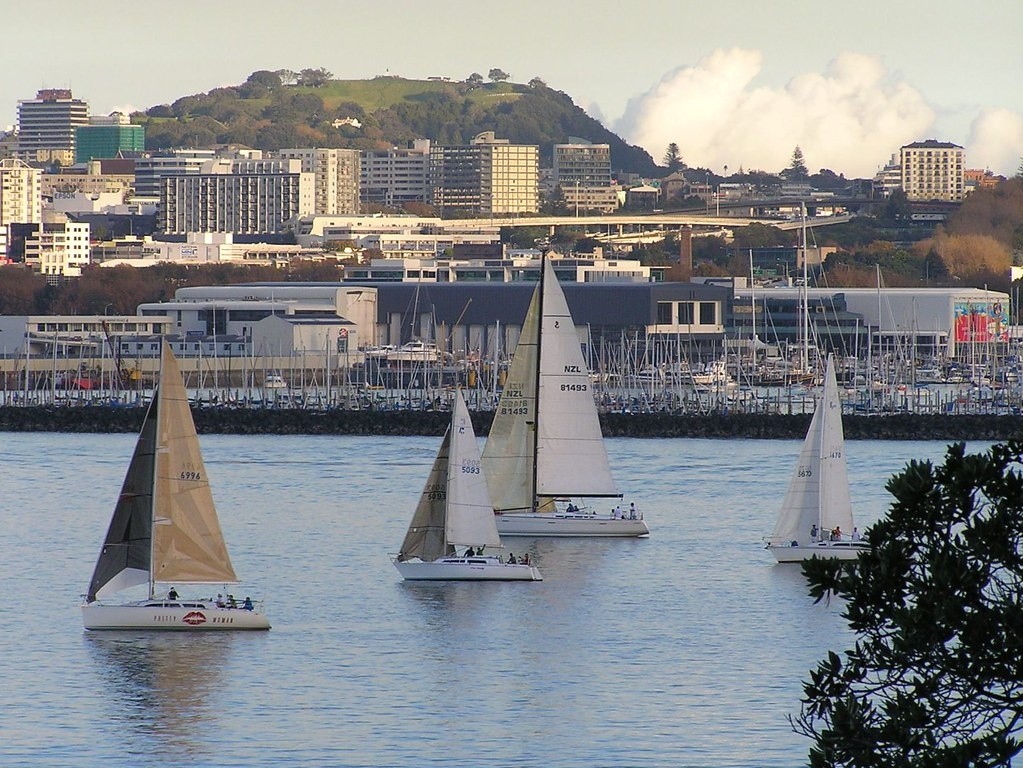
[851,527,860,542]
[861,532,871,542]
[476,544,486,556]
[519,553,531,566]
[464,546,475,557]
[506,552,516,564]
[810,524,822,543]
[629,502,638,520]
[566,503,579,512]
[168,586,180,607]
[615,506,625,519]
[241,597,254,611]
[217,594,226,608]
[610,509,616,520]
[226,593,237,608]
[830,525,842,541]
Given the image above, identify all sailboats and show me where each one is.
[763,352,883,564]
[474,250,650,538]
[82,330,274,632]
[0,203,1023,414]
[386,382,544,582]
